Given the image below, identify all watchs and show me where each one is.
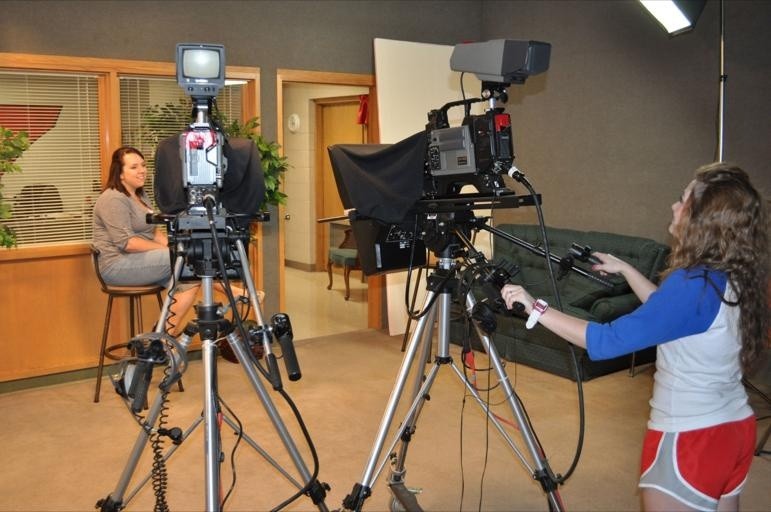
[525,298,549,329]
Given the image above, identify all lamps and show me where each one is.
[638,1,727,162]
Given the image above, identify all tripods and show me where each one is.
[94,277,331,512]
[341,259,565,512]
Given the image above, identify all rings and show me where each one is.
[508,288,514,297]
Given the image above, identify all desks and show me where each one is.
[0,211,93,244]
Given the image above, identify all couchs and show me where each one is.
[449,223,671,383]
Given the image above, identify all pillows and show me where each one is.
[569,281,631,309]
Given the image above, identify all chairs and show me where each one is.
[316,217,365,300]
[88,245,185,410]
[20,186,62,212]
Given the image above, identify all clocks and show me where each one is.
[287,114,300,133]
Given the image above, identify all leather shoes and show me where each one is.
[256,291,264,304]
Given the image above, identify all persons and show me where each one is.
[91,145,265,369]
[500,162,771,512]
